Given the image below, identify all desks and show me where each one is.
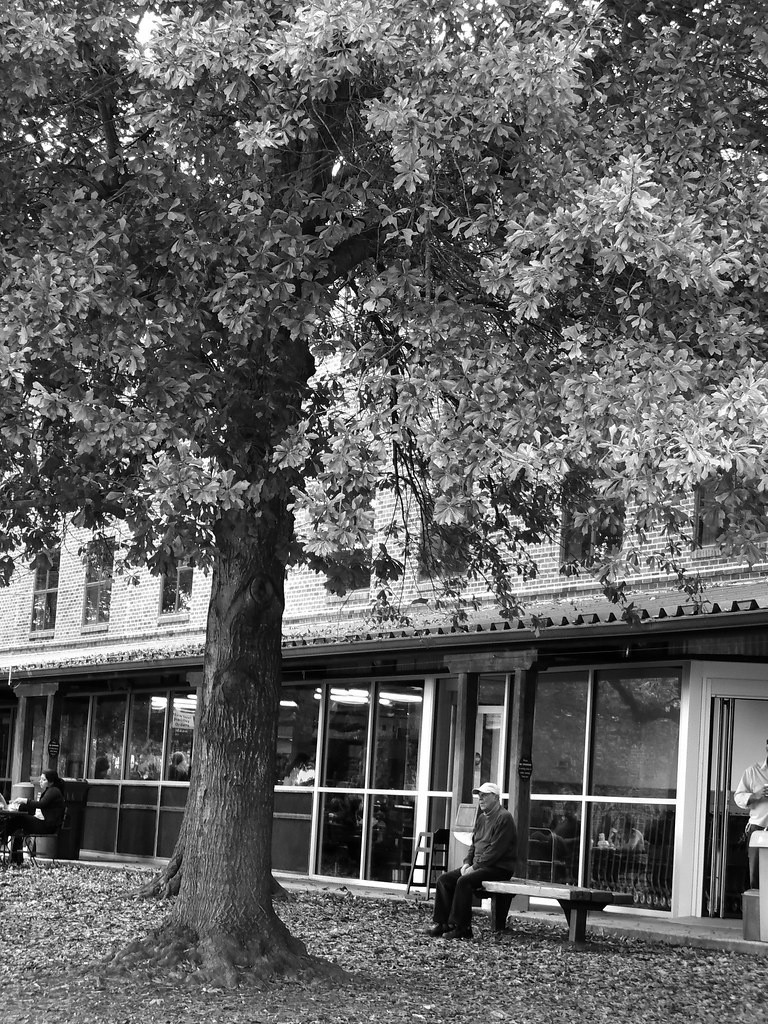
[592,847,650,891]
[0,811,28,867]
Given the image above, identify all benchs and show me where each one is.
[482,880,613,941]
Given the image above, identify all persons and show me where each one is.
[94,751,190,782]
[529,799,646,888]
[0,768,68,869]
[412,782,518,942]
[733,737,768,892]
[328,780,416,883]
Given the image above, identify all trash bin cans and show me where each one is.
[32,777,88,860]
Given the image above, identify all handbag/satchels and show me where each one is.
[738,824,755,846]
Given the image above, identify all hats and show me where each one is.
[472,782,501,796]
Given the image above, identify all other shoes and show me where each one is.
[428,926,474,939]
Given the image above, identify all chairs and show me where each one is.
[19,807,68,868]
[405,828,452,901]
[619,840,650,888]
